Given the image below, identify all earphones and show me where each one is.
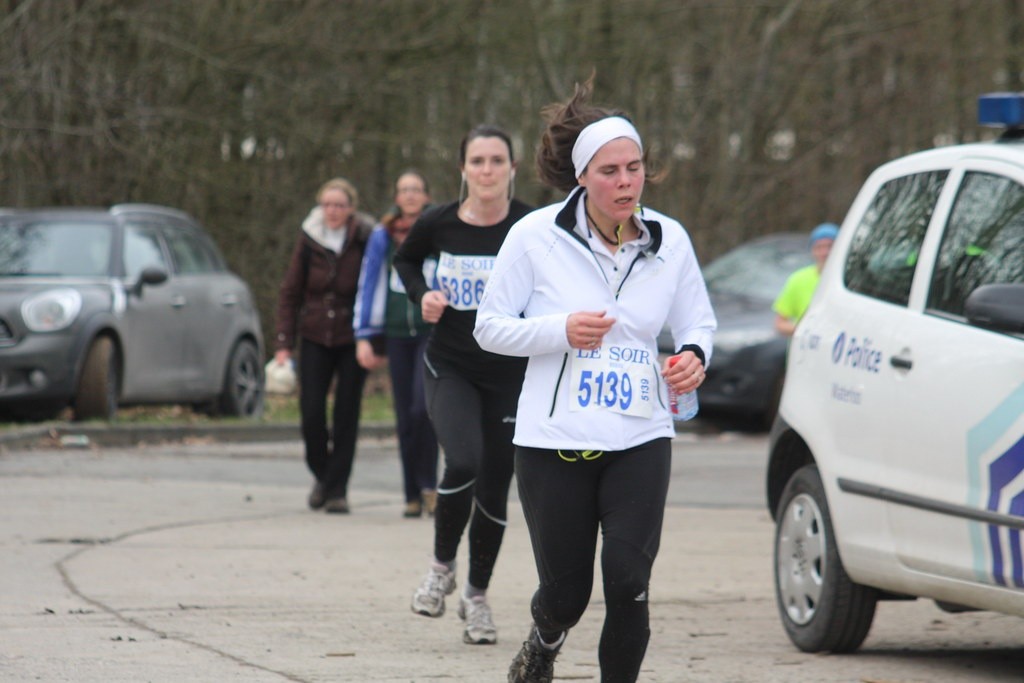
[510,170,515,179]
[462,172,466,180]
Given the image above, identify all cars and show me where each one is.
[764,89,1024,654]
[657,233,828,425]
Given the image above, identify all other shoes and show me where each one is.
[326,497,347,511]
[405,499,420,516]
[309,480,328,508]
[422,488,436,514]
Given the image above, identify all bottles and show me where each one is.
[667,355,698,422]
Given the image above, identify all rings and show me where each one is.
[695,372,701,381]
[589,343,596,346]
[592,336,595,342]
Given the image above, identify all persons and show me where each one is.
[771,223,842,335]
[473,67,718,683]
[352,167,440,517]
[392,123,536,644]
[266,178,377,513]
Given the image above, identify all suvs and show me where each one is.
[0,203,265,421]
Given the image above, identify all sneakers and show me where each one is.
[458,592,496,644]
[411,563,456,618]
[508,624,569,683]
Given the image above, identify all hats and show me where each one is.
[812,224,838,241]
